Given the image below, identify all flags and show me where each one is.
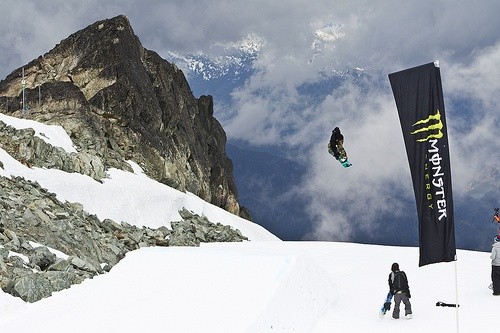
[388,60,458,267]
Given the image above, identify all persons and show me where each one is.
[490,235,500,295]
[388,263,412,320]
[330,126,347,162]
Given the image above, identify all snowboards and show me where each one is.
[379,289,394,316]
[328,149,353,168]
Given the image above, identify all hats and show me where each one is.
[495,234,500,241]
[392,263,399,270]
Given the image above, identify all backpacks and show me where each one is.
[393,272,406,290]
